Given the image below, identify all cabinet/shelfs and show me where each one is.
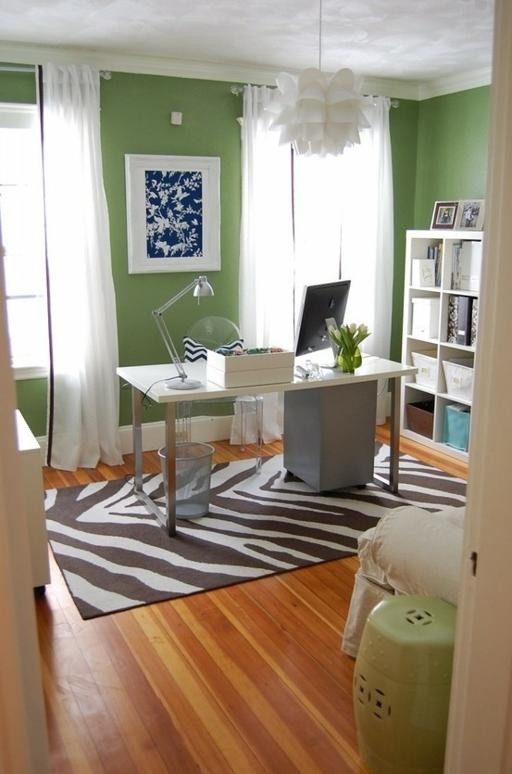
[10,406,52,603]
[391,226,484,465]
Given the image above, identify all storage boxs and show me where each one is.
[408,294,443,339]
[200,344,296,391]
[442,403,470,453]
[409,345,440,391]
[402,396,436,441]
[439,355,476,401]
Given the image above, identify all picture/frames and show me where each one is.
[428,199,459,230]
[123,151,223,274]
[451,196,485,231]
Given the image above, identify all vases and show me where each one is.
[336,344,364,374]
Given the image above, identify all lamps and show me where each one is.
[149,273,216,391]
[263,0,372,158]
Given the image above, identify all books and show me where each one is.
[426,239,462,291]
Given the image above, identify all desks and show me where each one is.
[114,339,418,540]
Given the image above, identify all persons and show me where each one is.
[439,207,452,224]
[465,206,475,226]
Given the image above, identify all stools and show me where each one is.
[341,502,467,659]
[350,594,463,774]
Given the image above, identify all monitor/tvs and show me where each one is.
[295,279,351,368]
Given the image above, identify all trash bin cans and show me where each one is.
[157,440,215,520]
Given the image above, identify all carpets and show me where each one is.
[33,436,467,624]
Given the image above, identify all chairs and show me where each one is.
[172,313,267,475]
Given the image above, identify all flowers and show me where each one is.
[326,319,375,374]
